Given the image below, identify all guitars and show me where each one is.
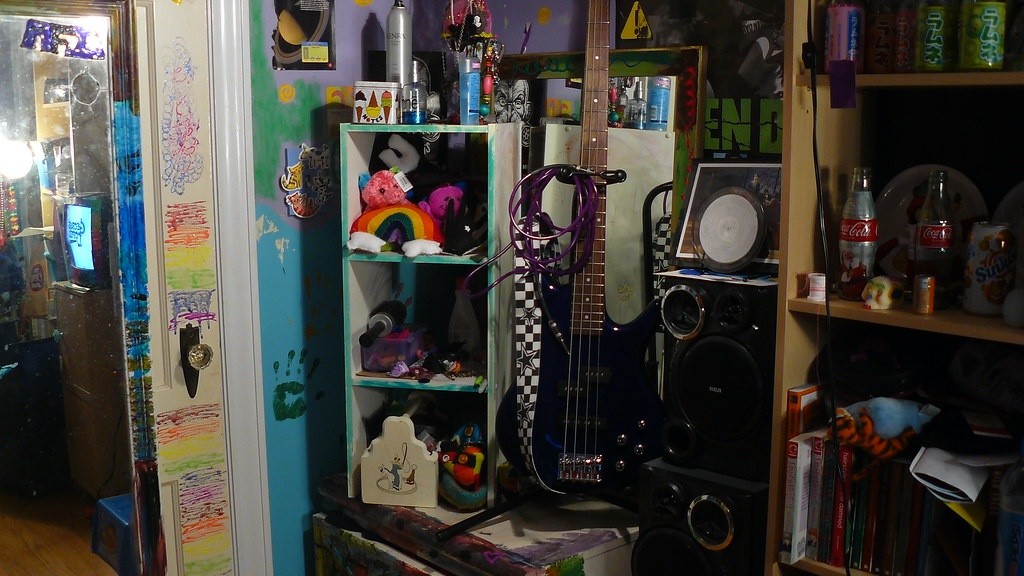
[494,0,664,495]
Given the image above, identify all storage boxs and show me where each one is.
[352,81,402,125]
[362,323,429,372]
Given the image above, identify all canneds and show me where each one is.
[913,274,935,314]
[401,82,427,125]
[962,220,1015,316]
[628,98,647,130]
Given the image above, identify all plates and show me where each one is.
[993,180,1024,289]
[874,163,989,294]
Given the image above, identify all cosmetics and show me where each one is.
[459,57,481,124]
[624,76,671,132]
[386,0,427,124]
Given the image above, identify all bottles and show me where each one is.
[624,81,646,129]
[481,61,494,95]
[447,277,480,356]
[459,58,480,125]
[914,168,956,308]
[997,440,1024,576]
[838,166,878,302]
[400,60,427,125]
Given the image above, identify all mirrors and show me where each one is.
[0,14,145,576]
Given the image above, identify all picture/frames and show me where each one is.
[667,158,783,278]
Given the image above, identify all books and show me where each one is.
[780,383,1024,575]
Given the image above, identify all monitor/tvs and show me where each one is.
[64,191,114,289]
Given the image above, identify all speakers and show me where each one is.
[632,456,769,576]
[660,274,779,483]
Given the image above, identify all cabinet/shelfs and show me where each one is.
[340,123,522,508]
[763,0,1024,576]
[312,484,640,576]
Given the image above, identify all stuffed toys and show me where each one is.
[829,396,940,459]
[346,133,488,258]
[348,135,470,257]
[438,421,487,512]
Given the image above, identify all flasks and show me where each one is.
[385,0,412,120]
